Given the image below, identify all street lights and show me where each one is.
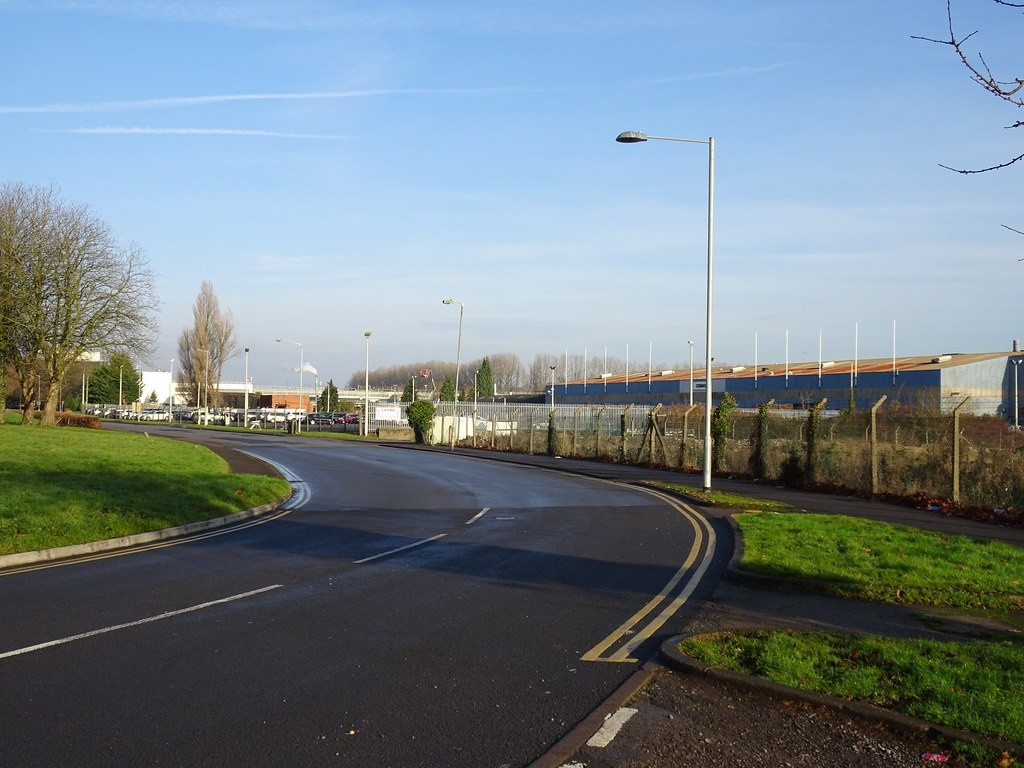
[168,359,175,424]
[687,340,696,407]
[443,299,464,449]
[363,331,372,438]
[244,347,249,428]
[549,365,557,412]
[196,349,210,426]
[275,338,304,433]
[615,130,716,495]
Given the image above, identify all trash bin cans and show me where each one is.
[288,420,296,435]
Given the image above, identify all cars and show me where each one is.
[87,403,366,423]
